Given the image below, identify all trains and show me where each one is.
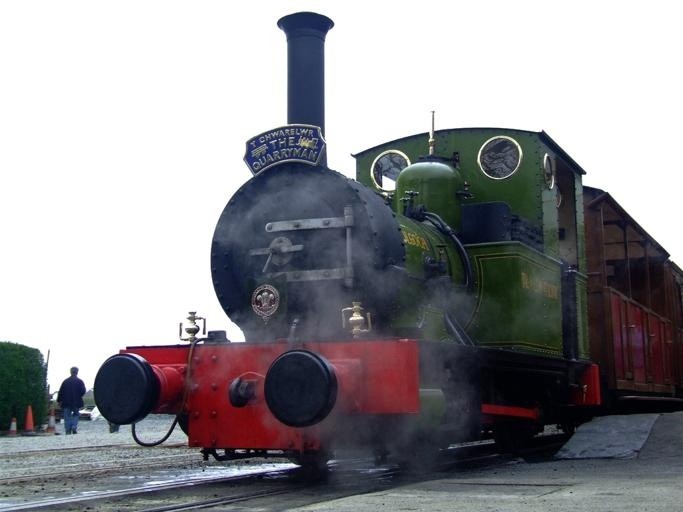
[92,8,682,481]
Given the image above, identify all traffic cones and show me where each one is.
[43,406,59,434]
[6,417,18,435]
[22,405,38,436]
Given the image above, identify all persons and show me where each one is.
[57,367,86,434]
